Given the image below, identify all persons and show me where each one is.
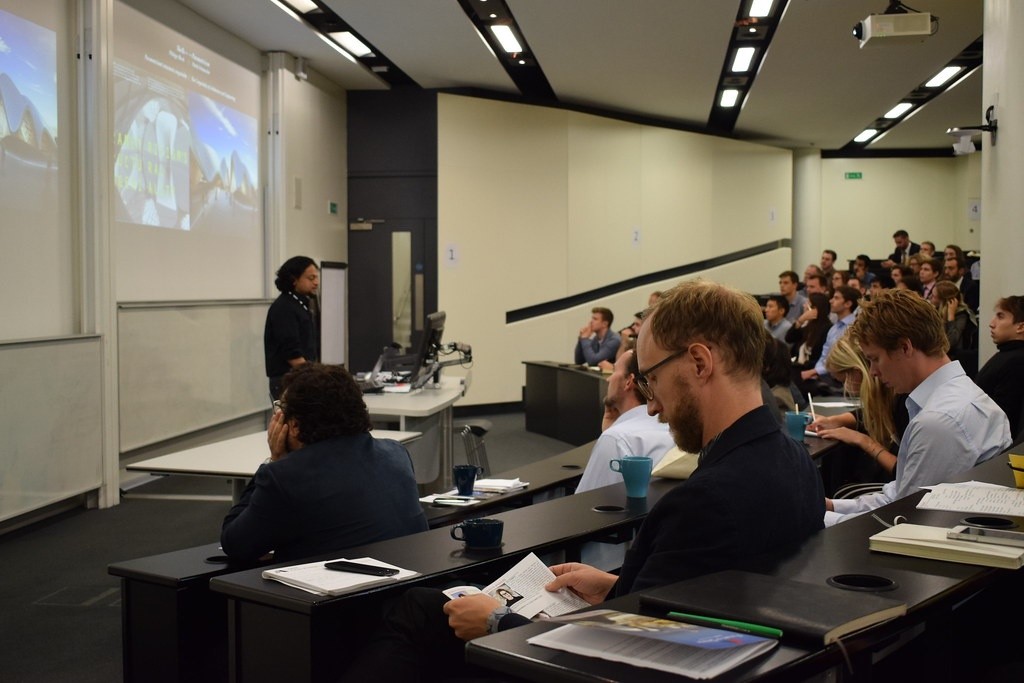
[574,229,980,413]
[824,289,1012,529]
[570,346,678,571]
[496,588,521,608]
[264,255,321,401]
[973,294,1024,448]
[805,330,910,500]
[442,278,826,642]
[220,364,431,567]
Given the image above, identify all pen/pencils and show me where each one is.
[666,612,783,638]
[808,392,819,433]
[808,304,812,311]
[434,498,467,503]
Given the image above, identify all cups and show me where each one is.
[1009,454,1024,488]
[785,411,813,441]
[609,457,654,498]
[450,519,503,546]
[453,464,484,496]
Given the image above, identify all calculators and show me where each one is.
[358,379,385,394]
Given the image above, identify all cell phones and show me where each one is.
[434,498,469,503]
[946,526,1024,548]
[324,561,399,577]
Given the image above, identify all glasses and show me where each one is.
[271,401,287,414]
[632,343,713,402]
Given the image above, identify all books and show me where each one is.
[638,568,905,644]
[868,522,1024,569]
[526,610,779,679]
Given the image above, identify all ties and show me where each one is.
[901,249,907,265]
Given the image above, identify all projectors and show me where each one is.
[851,13,932,49]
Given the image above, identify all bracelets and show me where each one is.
[874,447,888,460]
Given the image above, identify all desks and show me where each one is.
[109,359,1024,683]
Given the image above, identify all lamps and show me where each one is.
[945,120,998,146]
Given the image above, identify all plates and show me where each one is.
[462,543,505,551]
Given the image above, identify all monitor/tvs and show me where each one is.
[409,311,447,388]
